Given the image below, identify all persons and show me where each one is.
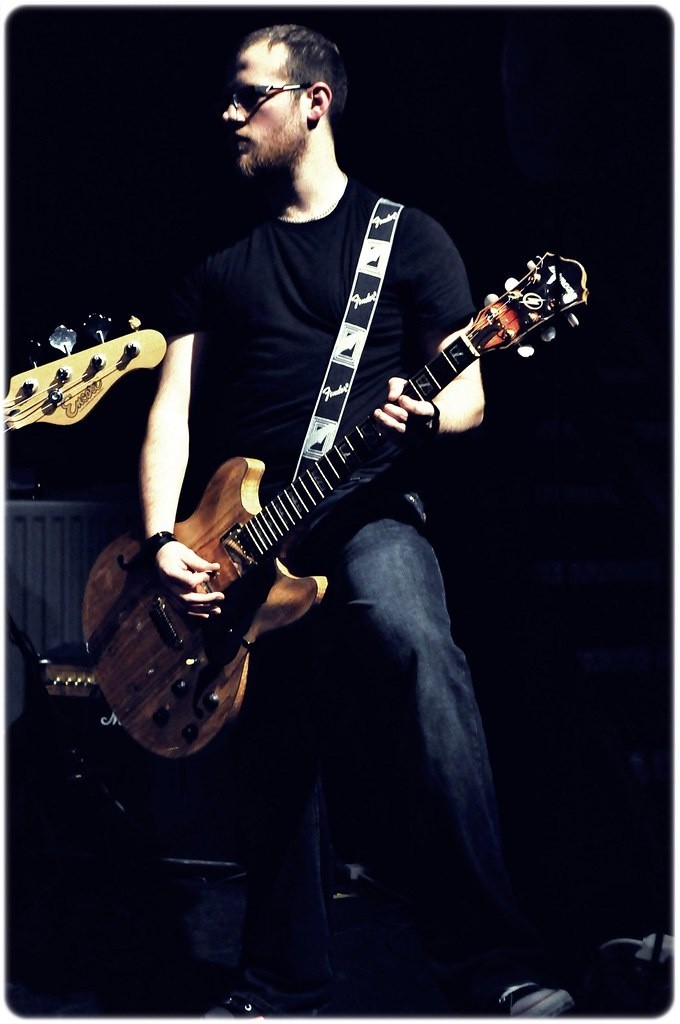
[139,26,577,1020]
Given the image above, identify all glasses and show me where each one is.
[223,83,313,112]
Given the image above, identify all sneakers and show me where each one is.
[500,982,574,1015]
[203,997,317,1017]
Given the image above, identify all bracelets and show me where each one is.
[141,531,177,559]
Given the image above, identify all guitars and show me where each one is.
[82,251,591,760]
[4,313,167,437]
